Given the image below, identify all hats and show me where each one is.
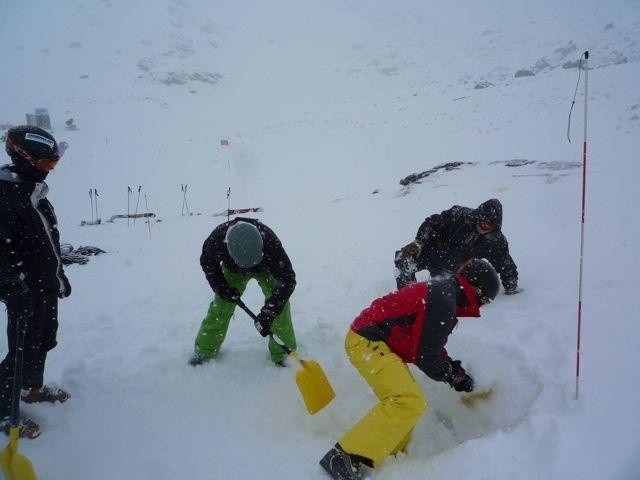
[226,221,265,271]
[456,257,502,302]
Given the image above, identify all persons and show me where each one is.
[0,124,74,443]
[317,258,505,480]
[187,216,298,368]
[388,196,522,311]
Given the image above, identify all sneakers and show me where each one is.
[318,447,361,480]
[0,415,42,441]
[20,384,71,405]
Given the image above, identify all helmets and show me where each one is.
[5,125,62,182]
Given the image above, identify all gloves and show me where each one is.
[400,238,425,259]
[449,359,472,393]
[215,284,242,304]
[253,305,281,338]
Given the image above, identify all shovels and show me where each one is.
[217,286,336,415]
[443,360,492,408]
[0,284,39,480]
